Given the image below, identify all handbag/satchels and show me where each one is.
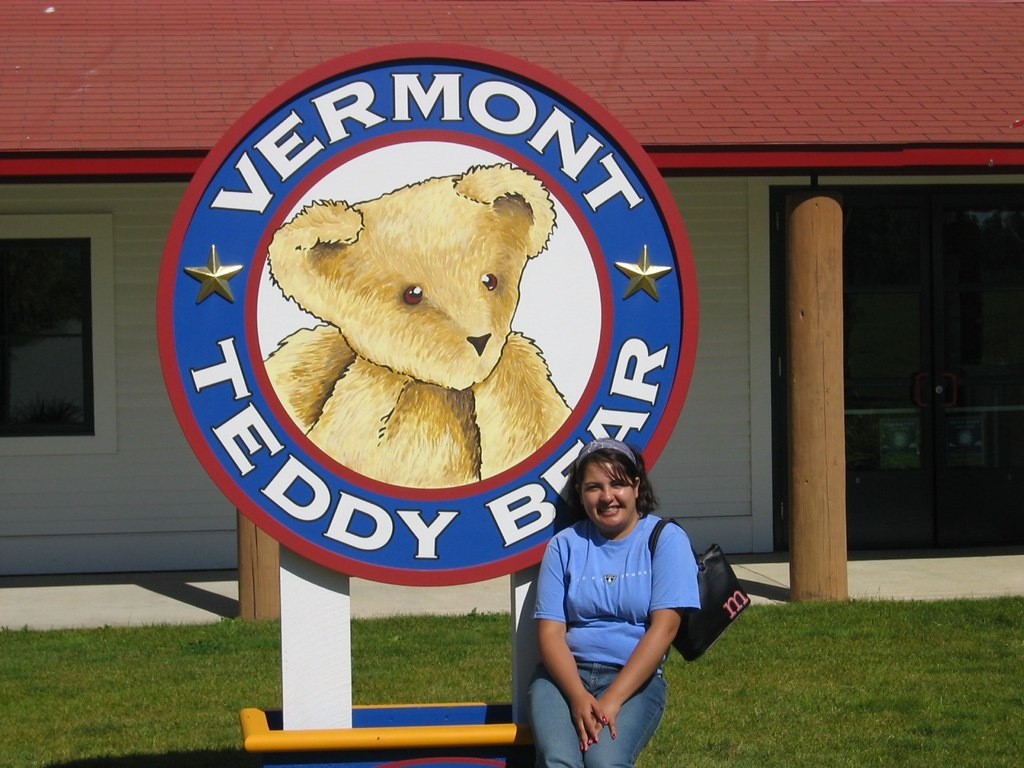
[649,516,750,662]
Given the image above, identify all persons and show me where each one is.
[525,438,702,768]
